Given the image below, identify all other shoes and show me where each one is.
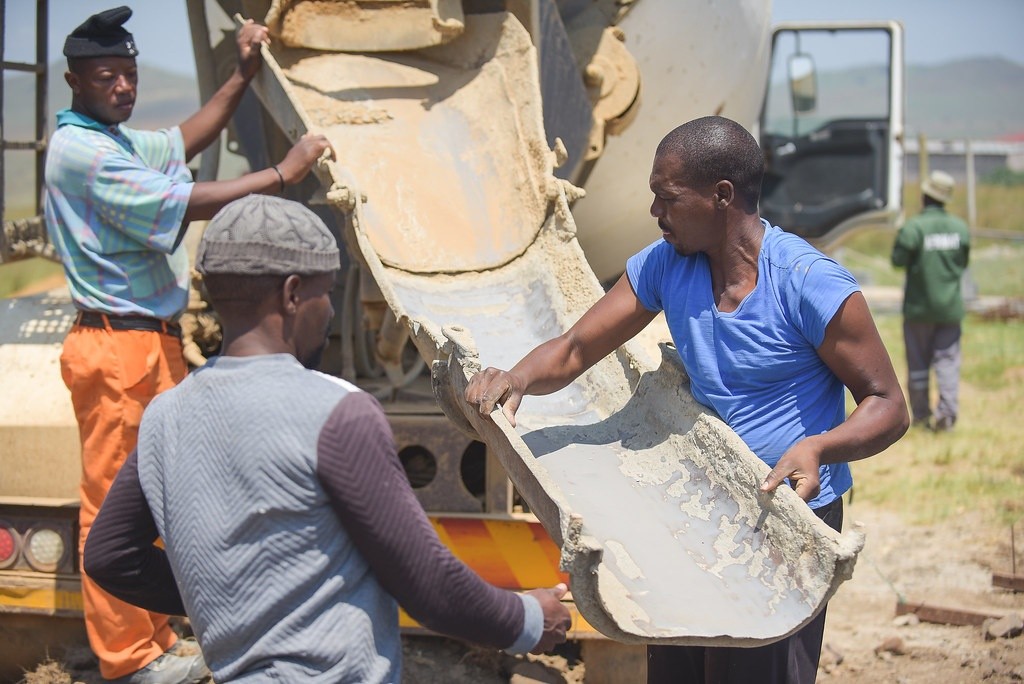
[110,653,210,684]
[165,638,204,656]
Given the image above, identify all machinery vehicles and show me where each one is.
[0,1,908,684]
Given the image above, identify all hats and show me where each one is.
[63,6,139,57]
[195,193,340,277]
[921,171,955,203]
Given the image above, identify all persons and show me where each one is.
[83,194,571,684]
[42,5,335,684]
[462,116,910,684]
[889,168,970,431]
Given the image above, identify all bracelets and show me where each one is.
[272,165,284,196]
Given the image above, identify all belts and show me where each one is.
[74,311,181,336]
